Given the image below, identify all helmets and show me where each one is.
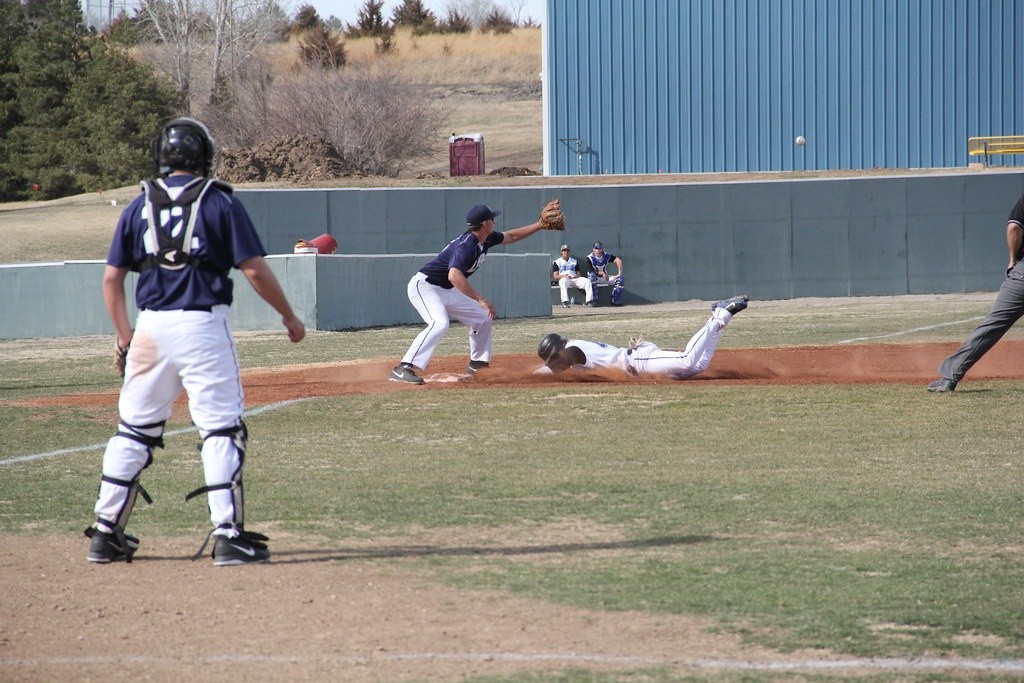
[538,334,565,365]
[155,116,213,174]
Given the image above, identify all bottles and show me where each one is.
[571,296,575,306]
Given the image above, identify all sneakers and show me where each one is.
[467,363,489,374]
[88,533,139,563]
[212,536,271,565]
[389,365,424,385]
[926,378,957,393]
[711,295,750,315]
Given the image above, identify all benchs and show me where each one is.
[551,283,609,289]
[967,134,1024,167]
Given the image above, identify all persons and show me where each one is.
[532,295,749,380]
[927,194,1024,393]
[553,244,594,308]
[388,199,565,385]
[586,241,625,306]
[83,117,307,566]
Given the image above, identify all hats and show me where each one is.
[560,245,572,251]
[593,241,603,249]
[467,205,502,225]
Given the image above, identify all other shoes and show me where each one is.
[562,302,570,308]
[610,298,624,307]
[584,301,595,307]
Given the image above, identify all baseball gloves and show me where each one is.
[540,197,566,232]
